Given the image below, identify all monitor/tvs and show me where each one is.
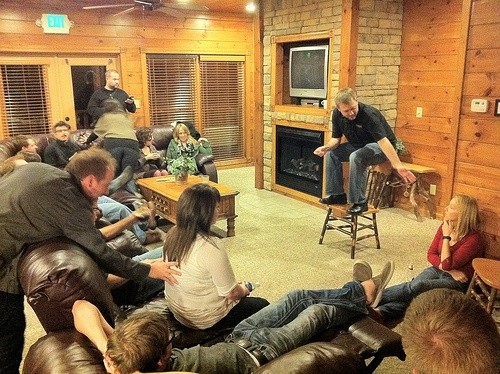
[290,45,328,105]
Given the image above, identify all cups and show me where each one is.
[132,199,150,225]
[313,103,319,109]
[321,100,328,109]
[200,175,210,183]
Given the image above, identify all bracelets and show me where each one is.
[442,236,450,240]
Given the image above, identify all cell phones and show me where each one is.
[127,95,134,101]
[246,281,255,290]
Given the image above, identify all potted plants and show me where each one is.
[167,155,198,182]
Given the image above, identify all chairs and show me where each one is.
[319,161,393,259]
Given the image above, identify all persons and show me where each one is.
[0,149,181,374]
[72,259,394,374]
[313,87,416,215]
[163,184,270,331]
[402,289,500,374]
[0,101,212,245]
[88,70,136,125]
[376,195,484,328]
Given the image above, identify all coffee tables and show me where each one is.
[136,172,240,238]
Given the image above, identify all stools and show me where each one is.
[465,257,499,315]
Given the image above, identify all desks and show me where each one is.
[369,160,437,222]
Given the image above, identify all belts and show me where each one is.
[232,336,269,366]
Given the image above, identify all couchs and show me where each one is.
[0,127,407,374]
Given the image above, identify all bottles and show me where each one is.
[243,281,260,293]
[150,144,158,154]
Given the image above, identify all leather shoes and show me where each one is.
[319,193,347,205]
[347,203,368,215]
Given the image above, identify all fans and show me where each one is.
[80,0,211,19]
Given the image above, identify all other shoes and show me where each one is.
[352,259,372,282]
[132,201,158,230]
[367,260,395,307]
[144,230,162,244]
